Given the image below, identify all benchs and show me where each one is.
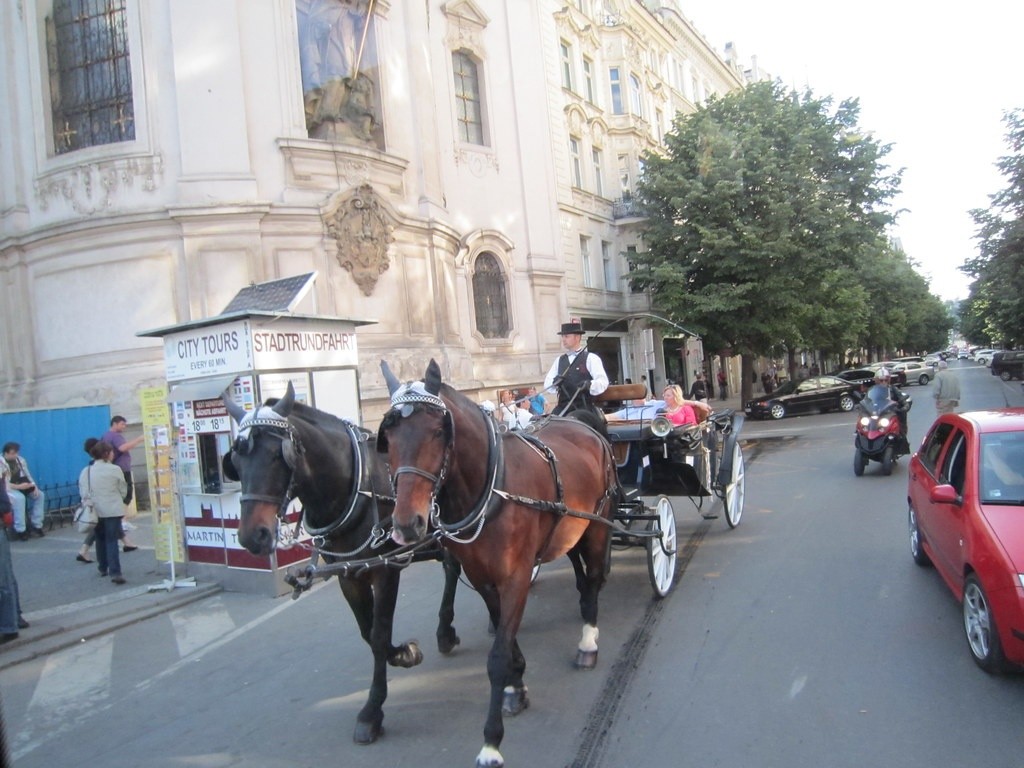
[591,384,654,442]
[622,400,714,454]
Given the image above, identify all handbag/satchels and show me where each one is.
[74,499,97,534]
[16,476,34,495]
[696,389,706,396]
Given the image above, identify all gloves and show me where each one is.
[578,379,591,392]
[552,375,566,387]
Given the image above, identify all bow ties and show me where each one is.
[567,351,579,355]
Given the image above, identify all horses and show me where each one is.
[375,358,624,768]
[221,381,461,746]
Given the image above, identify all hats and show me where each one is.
[557,323,586,334]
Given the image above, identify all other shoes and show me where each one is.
[17,612,29,630]
[1,630,21,643]
[16,529,28,540]
[101,571,107,576]
[112,577,126,584]
[31,524,44,536]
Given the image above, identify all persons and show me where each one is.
[932,360,961,414]
[1,442,44,540]
[76,435,139,563]
[498,390,517,428]
[544,323,609,418]
[79,441,128,583]
[625,361,862,404]
[526,386,549,415]
[508,396,534,429]
[873,368,910,413]
[99,415,146,530]
[660,384,698,428]
[0,453,29,646]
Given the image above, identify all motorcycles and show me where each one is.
[851,385,913,476]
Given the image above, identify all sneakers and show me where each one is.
[122,519,137,531]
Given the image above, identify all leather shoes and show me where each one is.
[76,553,94,564]
[123,544,138,553]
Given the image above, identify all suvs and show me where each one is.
[957,351,968,359]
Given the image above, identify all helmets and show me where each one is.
[873,367,891,380]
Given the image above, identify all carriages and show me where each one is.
[222,357,746,768]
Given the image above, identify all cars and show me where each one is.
[744,349,956,420]
[905,406,1024,671]
[969,347,1024,381]
